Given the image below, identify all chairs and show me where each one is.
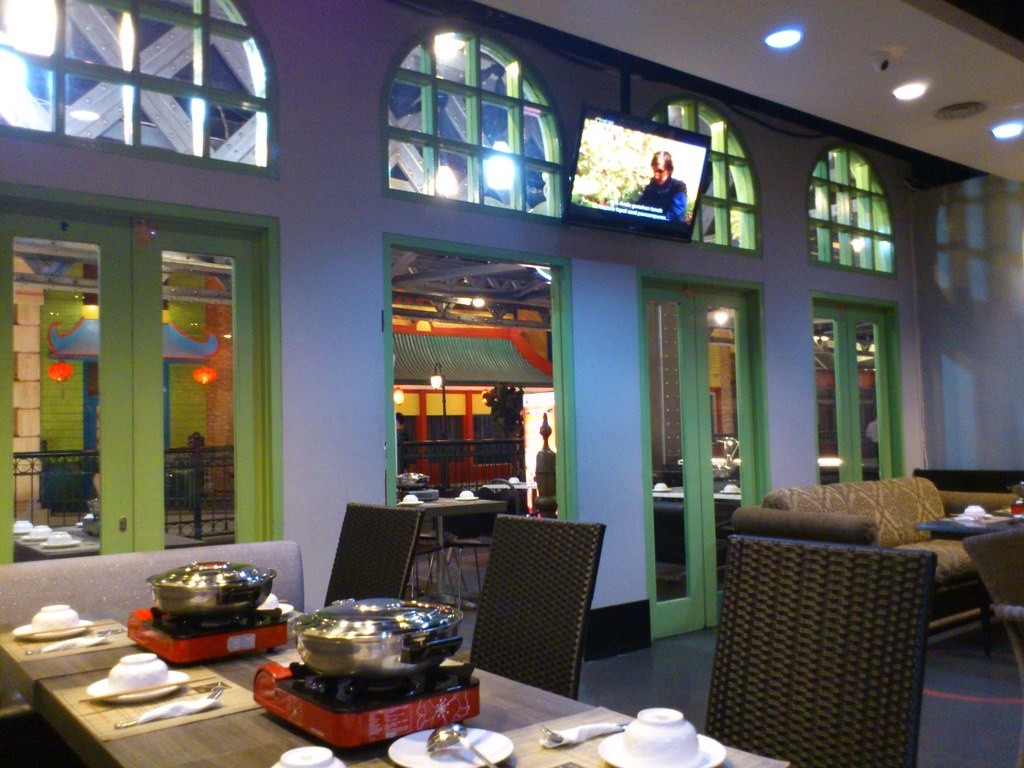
[963,529,1024,688]
[323,503,607,700]
[712,536,938,768]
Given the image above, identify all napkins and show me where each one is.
[137,698,223,725]
[39,637,106,653]
[538,723,621,749]
[954,515,984,523]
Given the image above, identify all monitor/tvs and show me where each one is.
[562,106,712,243]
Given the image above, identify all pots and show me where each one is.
[144,561,276,620]
[1007,482,1024,495]
[87,496,100,519]
[288,597,465,681]
[397,472,431,487]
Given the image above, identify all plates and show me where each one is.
[399,502,423,504]
[14,530,81,548]
[455,497,479,500]
[387,728,727,768]
[653,488,742,494]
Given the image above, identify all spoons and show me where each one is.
[540,722,632,743]
[78,654,222,729]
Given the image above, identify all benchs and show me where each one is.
[0,540,304,768]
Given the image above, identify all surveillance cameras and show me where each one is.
[873,53,889,72]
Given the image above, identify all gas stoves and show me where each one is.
[127,608,288,666]
[1011,497,1024,517]
[252,660,480,747]
[82,518,102,539]
[398,488,441,501]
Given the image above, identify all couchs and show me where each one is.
[729,475,992,640]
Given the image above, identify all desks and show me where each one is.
[13,525,207,560]
[652,485,739,507]
[397,499,508,609]
[483,484,538,517]
[915,510,1024,666]
[1,619,791,768]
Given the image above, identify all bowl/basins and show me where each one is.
[459,491,474,498]
[509,477,519,483]
[621,708,700,768]
[13,520,72,544]
[403,495,418,502]
[654,482,739,492]
[964,505,985,517]
[13,603,117,655]
[271,745,345,768]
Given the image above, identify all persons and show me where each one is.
[395,412,408,475]
[637,151,688,224]
